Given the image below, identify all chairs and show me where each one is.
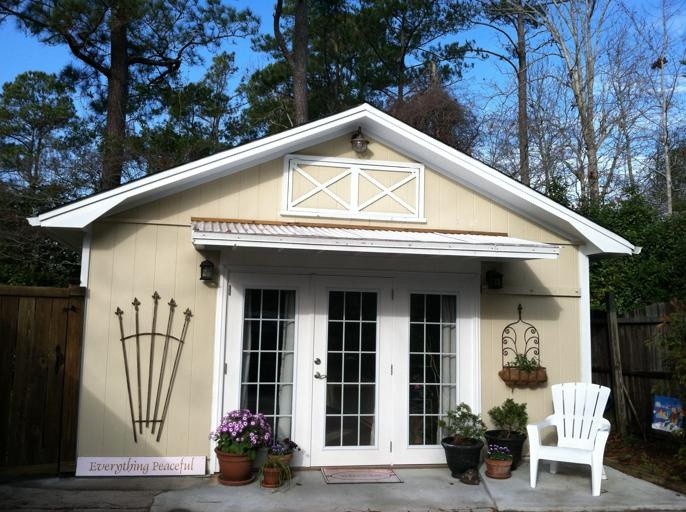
[527,381,612,497]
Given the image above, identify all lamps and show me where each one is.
[199,252,214,280]
[349,126,370,154]
[482,270,504,290]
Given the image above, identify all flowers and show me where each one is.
[207,408,300,459]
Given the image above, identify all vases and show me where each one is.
[214,446,293,488]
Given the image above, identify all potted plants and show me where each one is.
[435,398,529,479]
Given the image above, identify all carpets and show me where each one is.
[320,464,403,483]
